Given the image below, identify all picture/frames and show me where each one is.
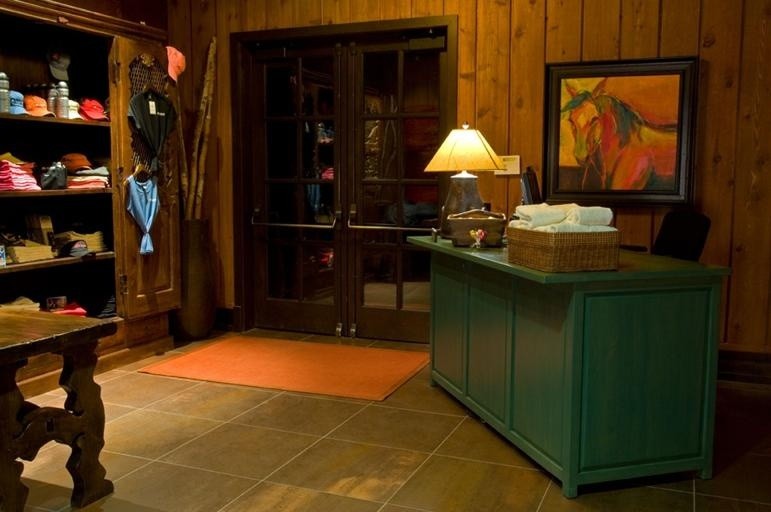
[541,58,700,215]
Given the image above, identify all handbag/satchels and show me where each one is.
[38,161,69,190]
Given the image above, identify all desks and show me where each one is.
[0,306,118,511]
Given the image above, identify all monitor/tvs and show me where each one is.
[519,166,541,204]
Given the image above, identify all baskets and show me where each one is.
[505,225,620,273]
[447,209,506,249]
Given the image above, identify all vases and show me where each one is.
[180,219,216,337]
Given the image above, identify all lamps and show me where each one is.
[423,120,508,239]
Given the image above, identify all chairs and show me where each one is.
[619,205,712,262]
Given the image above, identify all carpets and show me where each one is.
[138,332,430,403]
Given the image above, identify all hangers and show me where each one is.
[132,71,168,105]
[122,157,153,188]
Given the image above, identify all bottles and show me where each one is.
[0,70,10,114]
[25,80,69,120]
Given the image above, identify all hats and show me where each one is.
[62,152,93,171]
[26,95,57,118]
[80,98,110,122]
[61,240,97,259]
[8,91,28,116]
[165,45,187,85]
[68,100,84,121]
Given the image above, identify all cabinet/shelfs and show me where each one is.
[406,235,733,498]
[0,1,182,394]
